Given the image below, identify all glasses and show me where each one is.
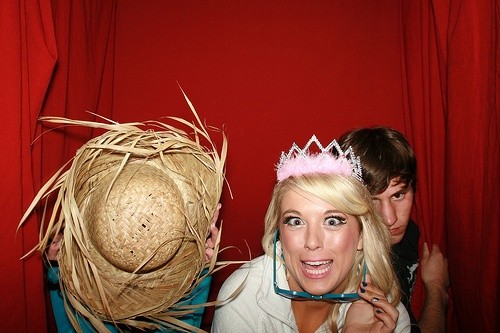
[272,228,367,303]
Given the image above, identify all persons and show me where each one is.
[47,199,221,269]
[209,134,411,333]
[334,127,448,333]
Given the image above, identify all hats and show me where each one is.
[15,79,253,333]
